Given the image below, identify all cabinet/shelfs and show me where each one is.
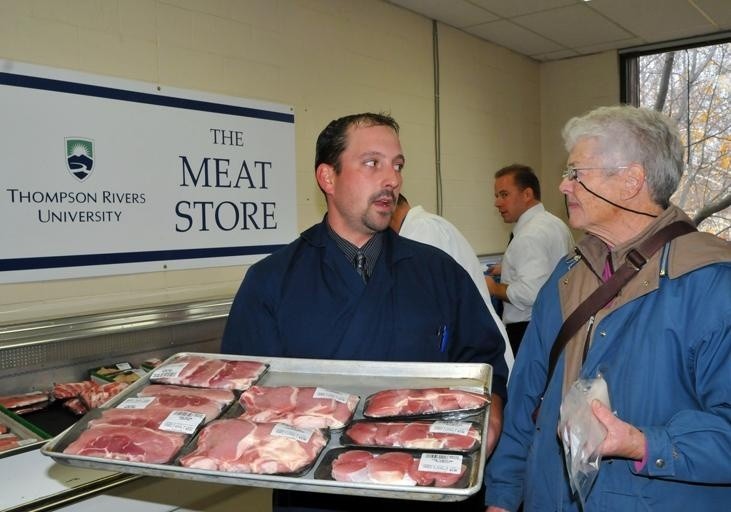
[1,248,504,512]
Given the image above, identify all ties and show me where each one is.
[353,252,369,284]
[507,232,514,247]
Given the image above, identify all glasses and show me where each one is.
[561,165,630,180]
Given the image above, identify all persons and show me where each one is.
[387,191,515,395]
[220,113,508,512]
[485,164,576,359]
[485,105,731,512]
